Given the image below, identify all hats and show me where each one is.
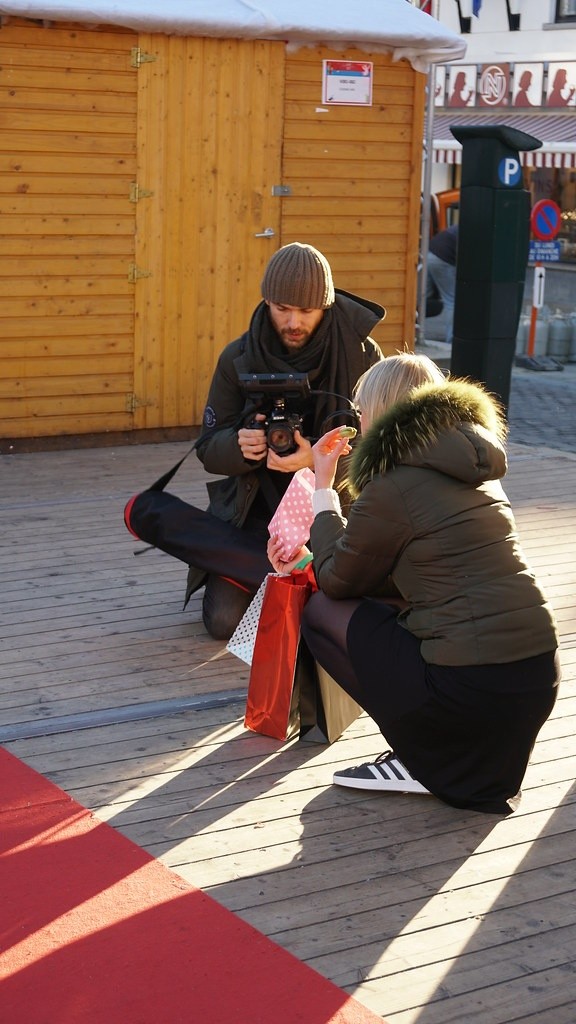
[261,242,335,310]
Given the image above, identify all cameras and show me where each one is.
[246,410,303,458]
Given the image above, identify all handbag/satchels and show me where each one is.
[226,553,365,744]
[123,486,277,590]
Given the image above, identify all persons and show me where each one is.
[427,224,462,342]
[266,340,562,815]
[181,239,389,640]
